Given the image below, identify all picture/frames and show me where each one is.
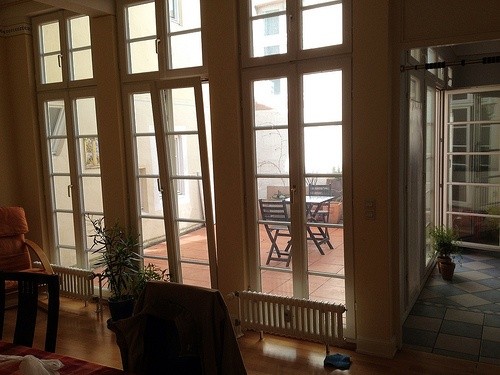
[84,138,100,169]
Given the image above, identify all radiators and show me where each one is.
[226,289,346,346]
[33,260,95,301]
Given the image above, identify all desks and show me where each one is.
[283,196,334,255]
[0,342,127,375]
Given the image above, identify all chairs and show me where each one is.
[108,280,248,375]
[0,269,60,353]
[0,206,54,308]
[307,182,332,243]
[259,198,293,268]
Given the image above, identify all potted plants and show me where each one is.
[83,210,174,322]
[428,224,463,280]
[269,191,291,218]
[320,184,342,224]
